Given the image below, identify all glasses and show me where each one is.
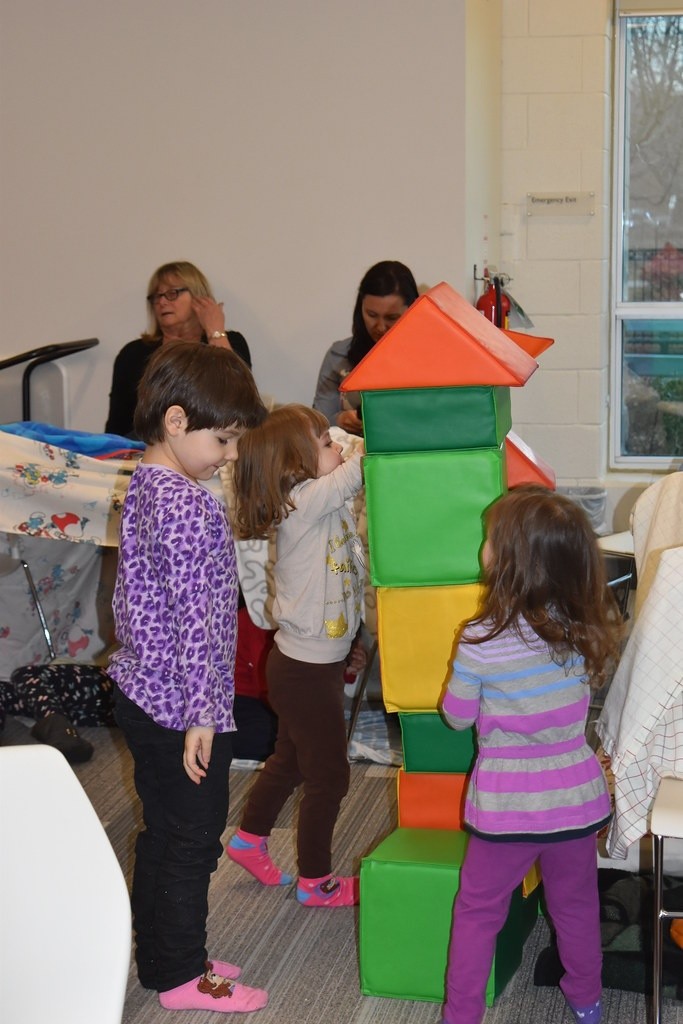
[147,287,189,304]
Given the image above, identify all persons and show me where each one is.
[312,261,420,437]
[441,482,627,1024]
[104,261,252,442]
[225,403,364,909]
[105,339,269,1011]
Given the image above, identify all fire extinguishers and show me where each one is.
[476,272,512,331]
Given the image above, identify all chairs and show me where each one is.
[594,467,683,1024]
[596,486,647,614]
[0,744,132,1024]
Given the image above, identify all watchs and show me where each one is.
[207,331,228,338]
